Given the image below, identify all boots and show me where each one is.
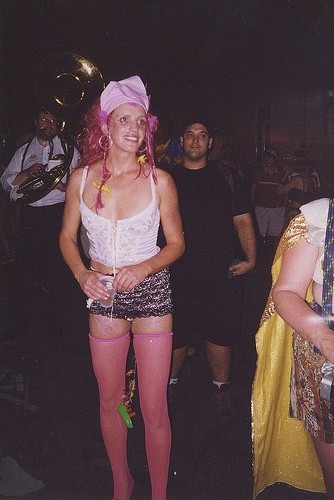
[210,382,239,426]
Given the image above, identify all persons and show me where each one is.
[157,120,255,417]
[59,76,185,500]
[250,149,320,242]
[0,110,80,293]
[251,198,334,500]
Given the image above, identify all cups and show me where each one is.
[100,277,115,308]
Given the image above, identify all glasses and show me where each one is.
[36,117,58,126]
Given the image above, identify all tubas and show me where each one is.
[8,52,107,205]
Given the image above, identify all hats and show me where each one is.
[99,75,151,136]
